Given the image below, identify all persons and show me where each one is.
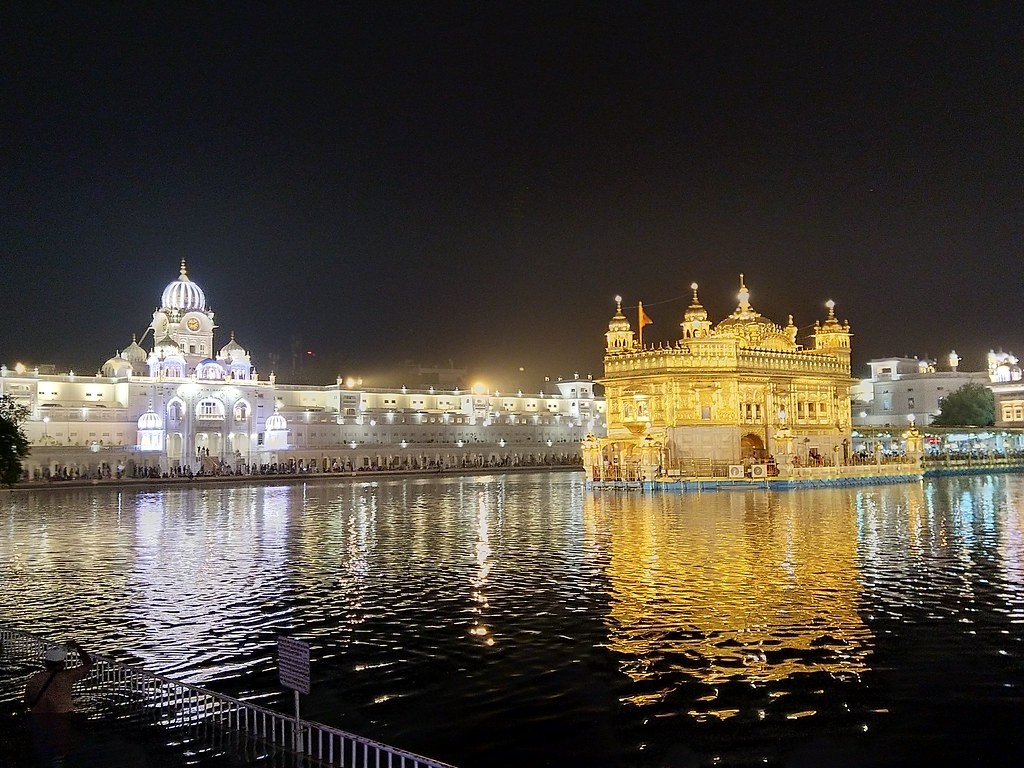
[852,440,1024,461]
[18,446,583,482]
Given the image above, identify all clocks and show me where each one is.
[187,318,200,331]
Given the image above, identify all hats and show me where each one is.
[45,645,68,661]
